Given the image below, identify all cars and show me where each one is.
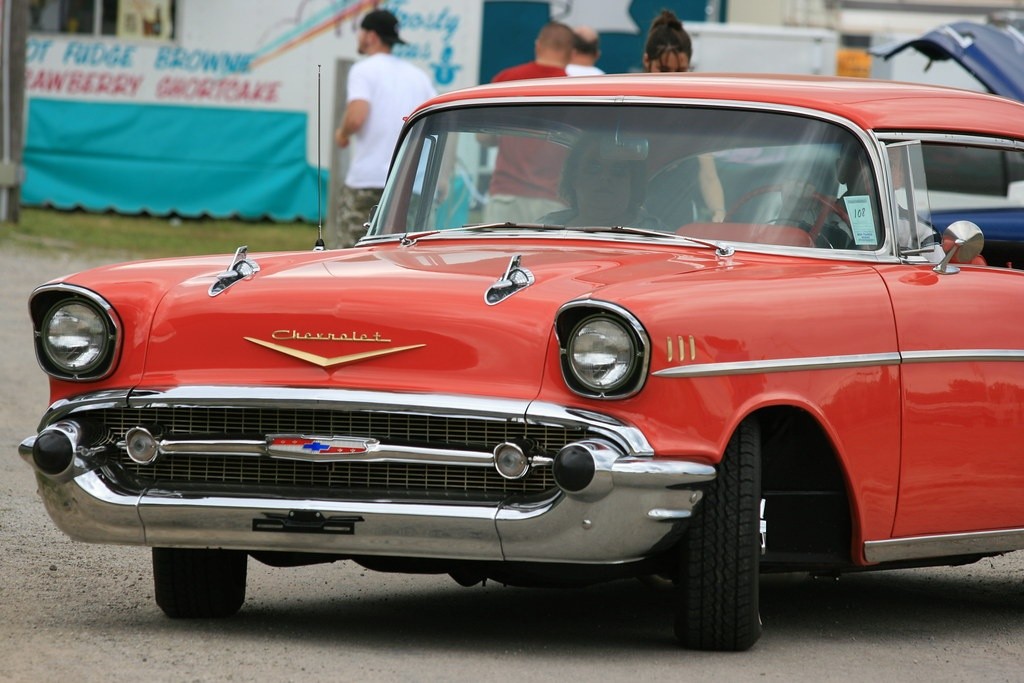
[628,20,1024,273]
[19,68,1023,654]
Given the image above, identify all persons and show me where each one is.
[774,133,944,271]
[327,6,727,263]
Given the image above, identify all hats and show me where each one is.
[361,10,404,44]
